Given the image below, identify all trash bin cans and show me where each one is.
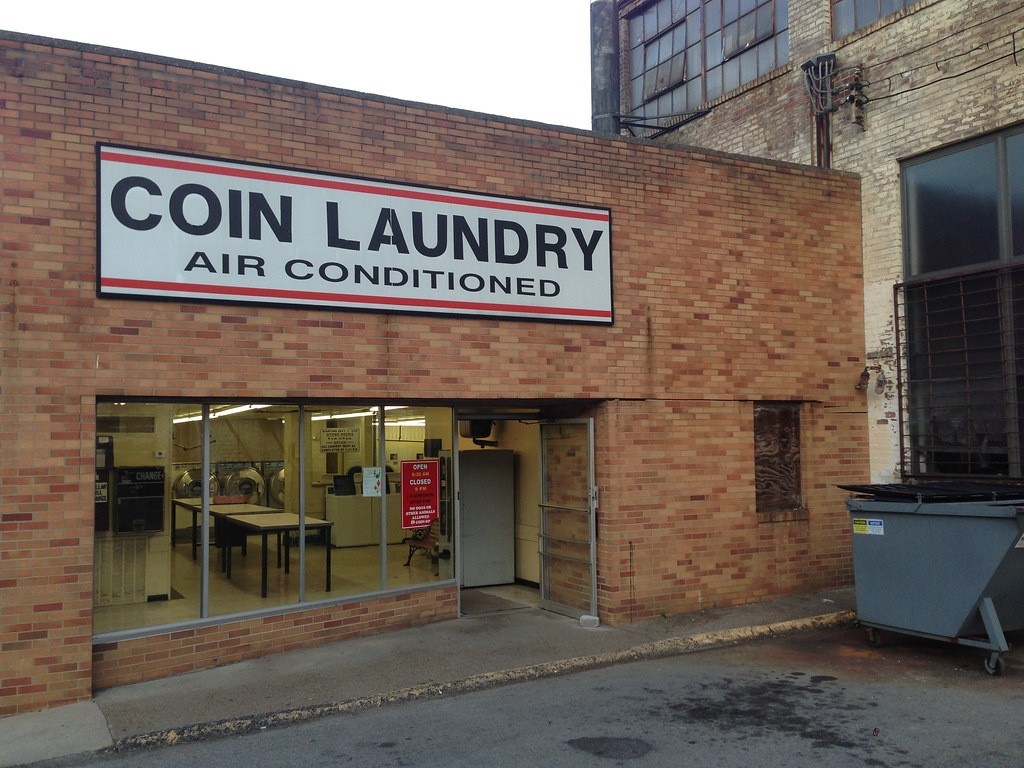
[833,481,1024,675]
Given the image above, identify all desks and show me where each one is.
[225,512,334,598]
[192,503,286,573]
[171,497,248,556]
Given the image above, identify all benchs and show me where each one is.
[402,522,439,576]
